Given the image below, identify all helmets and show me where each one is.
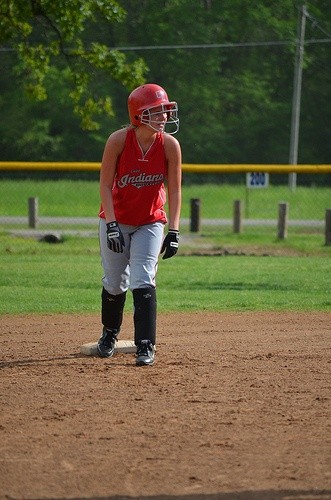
[127,84,175,125]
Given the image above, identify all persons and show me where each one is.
[98,83,183,366]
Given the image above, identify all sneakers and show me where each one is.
[97,325,119,358]
[136,339,157,366]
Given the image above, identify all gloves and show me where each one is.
[106,221,125,253]
[160,229,179,260]
